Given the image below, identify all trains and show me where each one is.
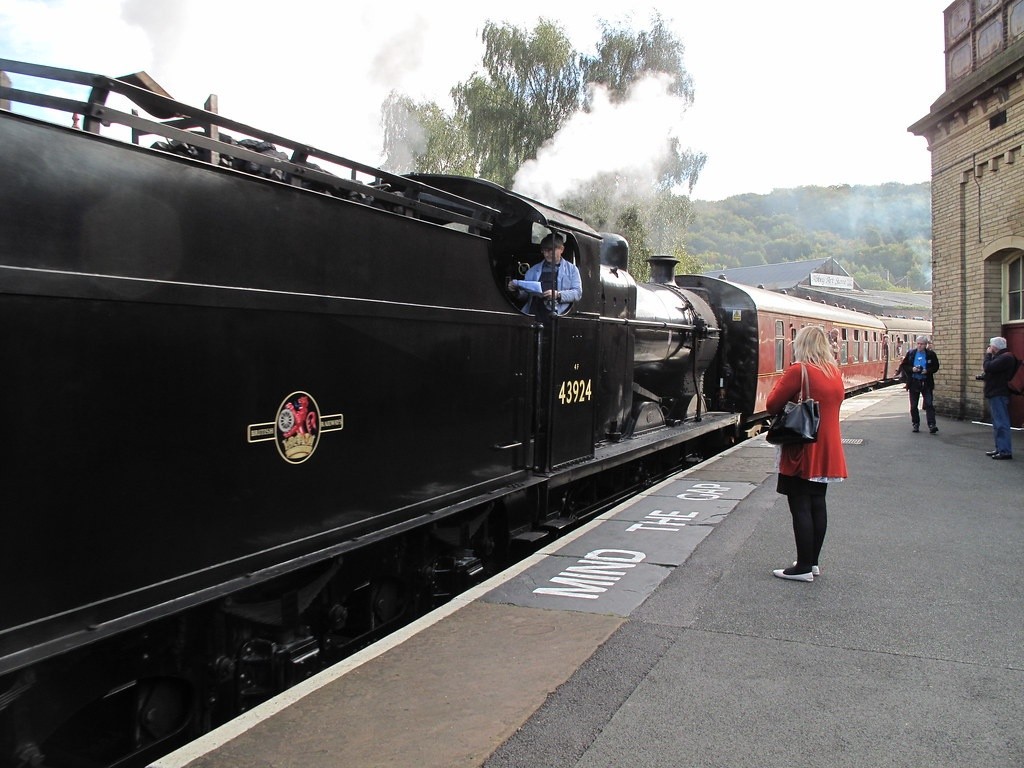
[0,53,932,768]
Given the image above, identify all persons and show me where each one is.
[894,356,909,391]
[507,232,583,316]
[766,326,847,582]
[903,336,939,433]
[828,328,840,350]
[976,337,1021,460]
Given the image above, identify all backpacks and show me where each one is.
[1001,352,1024,395]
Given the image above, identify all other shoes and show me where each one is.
[912,423,919,432]
[929,424,939,433]
[773,569,814,582]
[986,450,999,456]
[792,560,820,575]
[992,454,1012,460]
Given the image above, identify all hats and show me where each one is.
[540,233,563,249]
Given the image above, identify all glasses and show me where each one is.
[539,248,559,255]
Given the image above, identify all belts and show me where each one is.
[912,378,927,382]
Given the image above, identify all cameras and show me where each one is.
[916,365,923,374]
[976,373,986,380]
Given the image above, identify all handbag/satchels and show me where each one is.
[766,362,821,444]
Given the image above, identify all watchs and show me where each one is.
[558,291,561,298]
